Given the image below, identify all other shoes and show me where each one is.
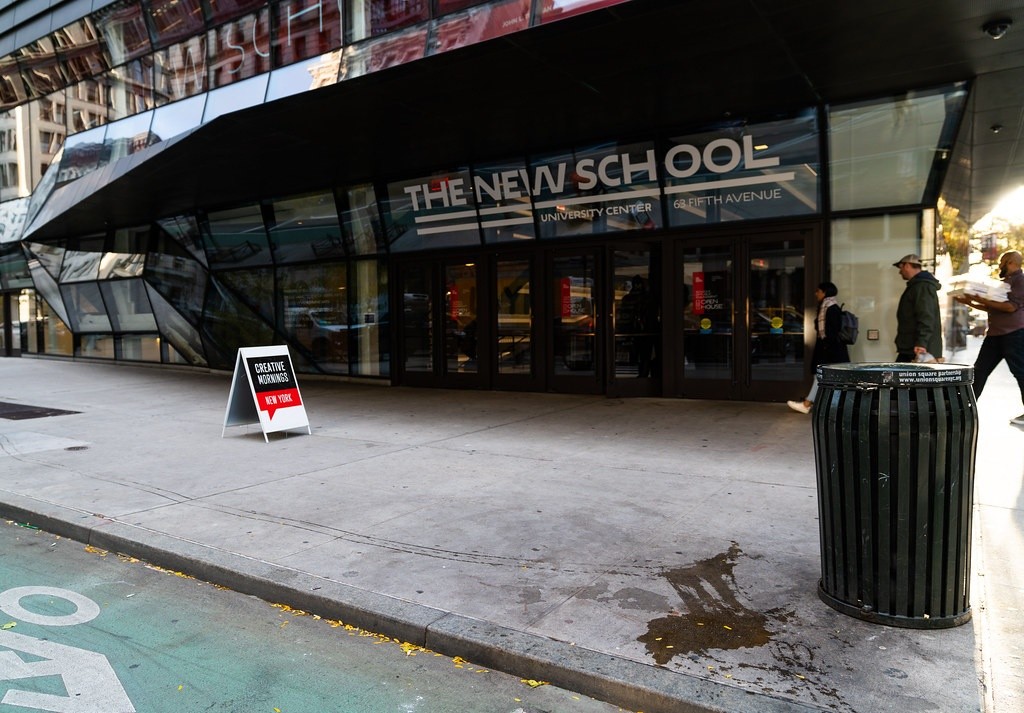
[1012,414,1024,426]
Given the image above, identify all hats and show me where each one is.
[892,253,922,268]
[817,280,838,297]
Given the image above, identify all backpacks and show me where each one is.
[840,303,858,345]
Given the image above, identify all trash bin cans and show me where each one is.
[810,359,980,630]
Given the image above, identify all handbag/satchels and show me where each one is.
[914,353,937,363]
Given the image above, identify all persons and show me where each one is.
[891,254,943,364]
[786,280,850,415]
[956,252,1024,428]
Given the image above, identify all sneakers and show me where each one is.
[786,399,811,414]
